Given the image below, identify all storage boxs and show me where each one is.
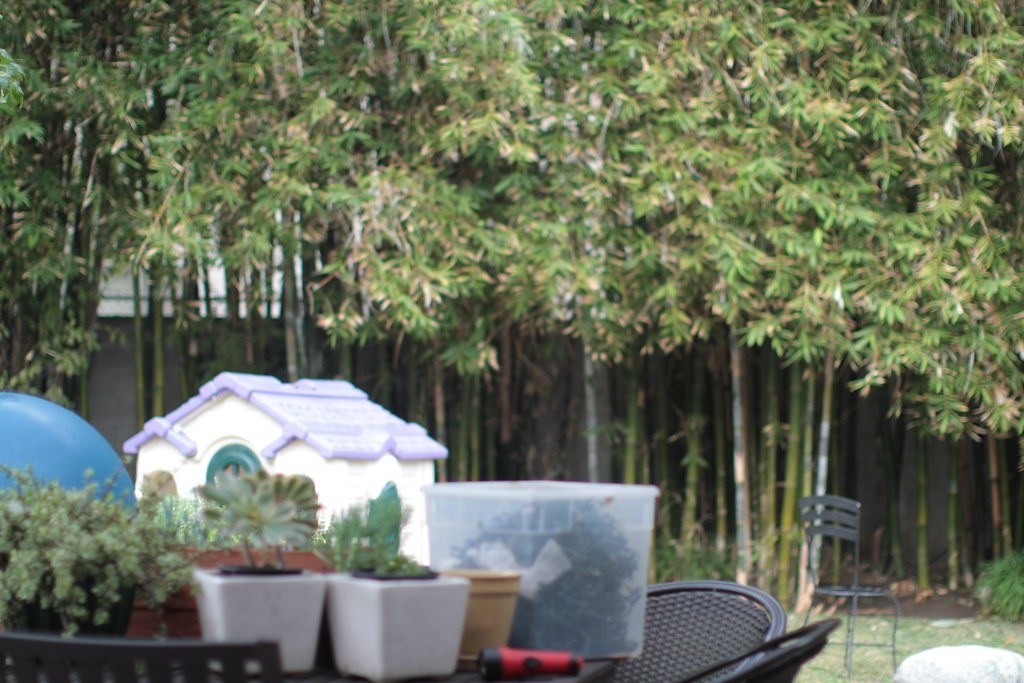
[419,479,662,662]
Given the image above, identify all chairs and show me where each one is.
[677,618,842,683]
[608,580,782,683]
[793,494,899,674]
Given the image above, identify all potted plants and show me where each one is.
[0,455,468,678]
